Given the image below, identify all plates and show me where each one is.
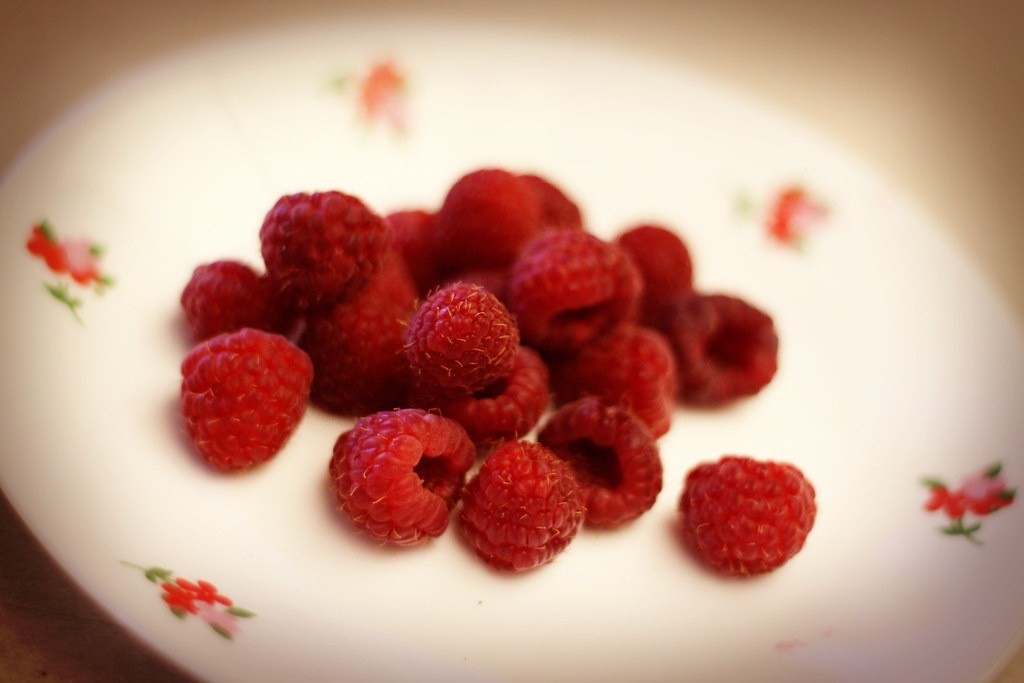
[2,19,1023,682]
